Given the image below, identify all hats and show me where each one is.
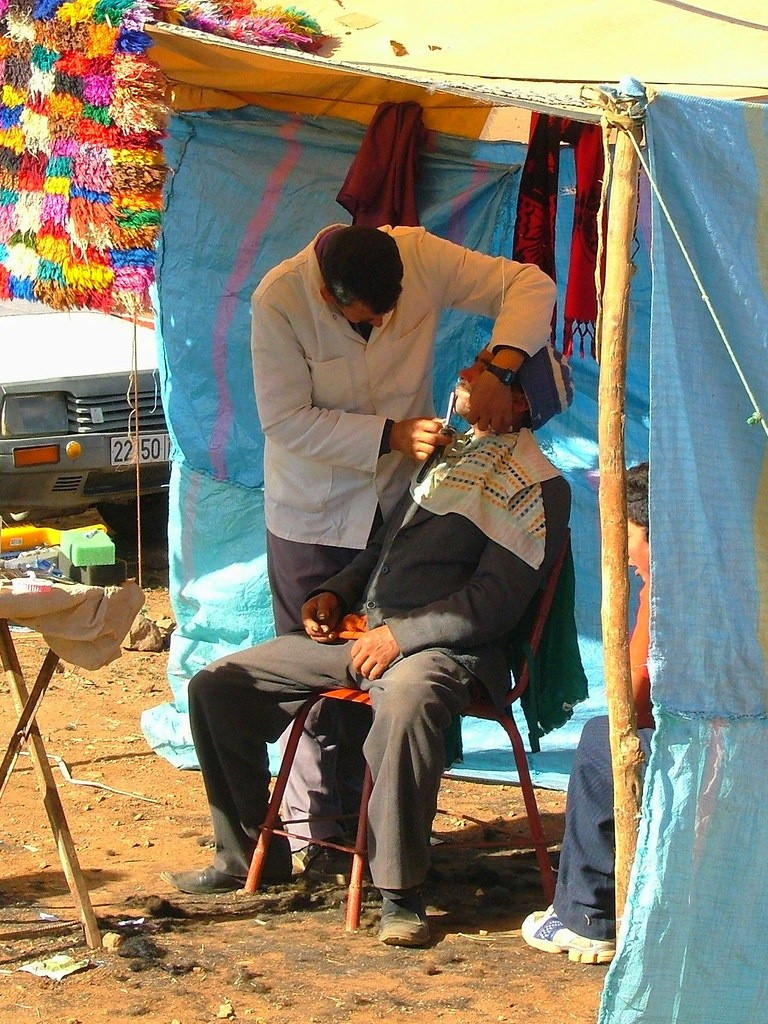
[518,345,575,431]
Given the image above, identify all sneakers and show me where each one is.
[292,836,354,885]
[521,904,616,964]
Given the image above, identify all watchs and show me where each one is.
[487,364,515,385]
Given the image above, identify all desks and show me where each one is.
[0,579,146,950]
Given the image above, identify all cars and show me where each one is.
[0,289,174,538]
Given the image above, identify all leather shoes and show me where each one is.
[161,866,293,895]
[382,897,431,946]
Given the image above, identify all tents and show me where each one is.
[1,0,767,1024]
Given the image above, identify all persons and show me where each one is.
[521,462,655,964]
[160,344,574,946]
[251,224,559,883]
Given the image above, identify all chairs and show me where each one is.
[242,525,571,931]
